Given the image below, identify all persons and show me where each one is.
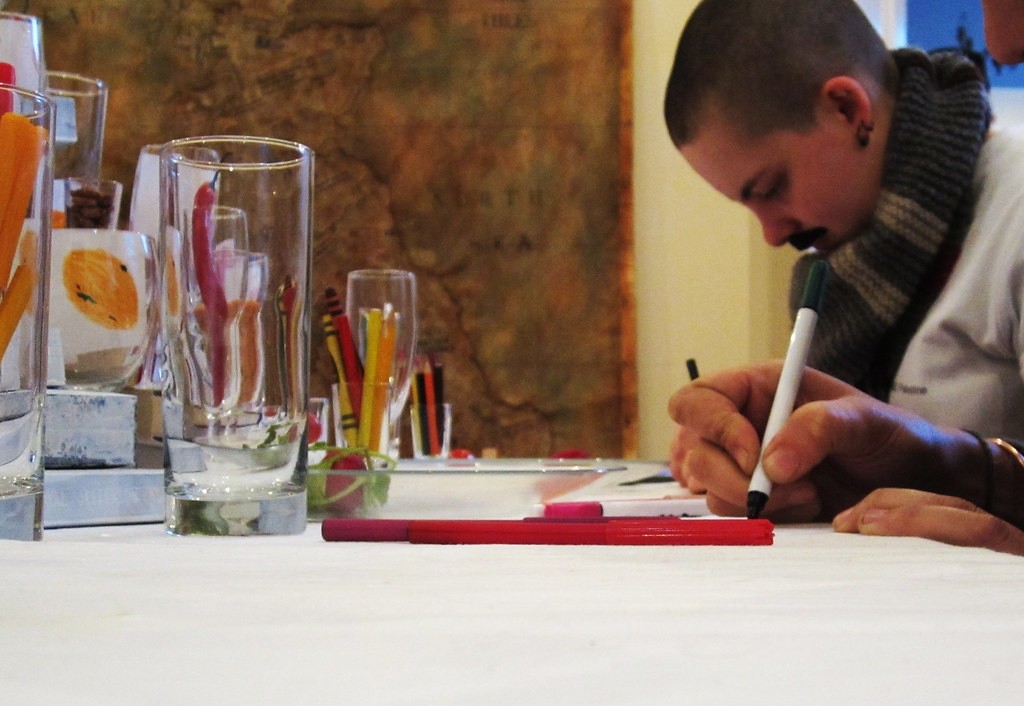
[664,1,1024,562]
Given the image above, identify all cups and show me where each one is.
[214,205,250,425]
[0,82,57,541]
[332,382,393,468]
[128,143,221,390]
[344,269,417,425]
[238,252,264,405]
[408,404,451,458]
[63,177,123,230]
[157,135,315,535]
[44,70,117,179]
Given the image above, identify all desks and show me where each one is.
[0,508,1024,705]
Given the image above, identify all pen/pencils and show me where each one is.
[322,518,775,547]
[687,358,699,383]
[534,500,707,519]
[322,288,400,450]
[411,354,445,458]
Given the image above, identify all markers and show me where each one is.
[747,307,818,519]
